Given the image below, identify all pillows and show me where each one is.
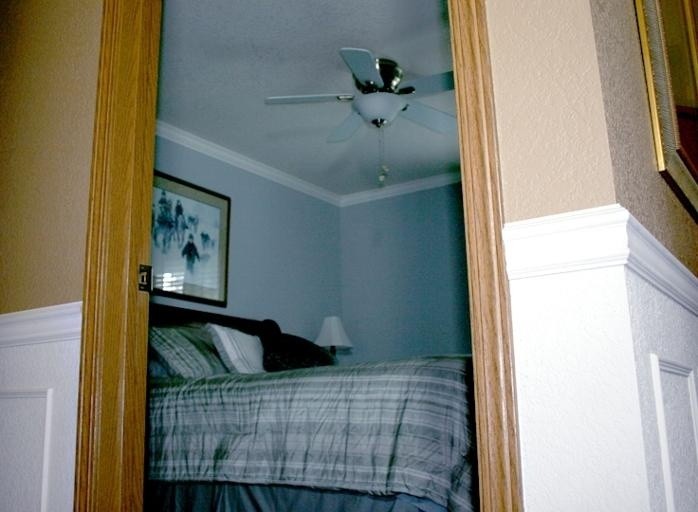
[203,321,266,375]
[147,357,173,378]
[149,321,230,378]
[255,319,335,373]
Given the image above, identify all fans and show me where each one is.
[262,43,458,145]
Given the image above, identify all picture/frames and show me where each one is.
[147,166,233,310]
[631,1,697,221]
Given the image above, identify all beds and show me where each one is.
[146,299,479,511]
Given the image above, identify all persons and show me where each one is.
[152,190,210,276]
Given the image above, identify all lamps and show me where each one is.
[350,87,409,132]
[314,314,353,361]
